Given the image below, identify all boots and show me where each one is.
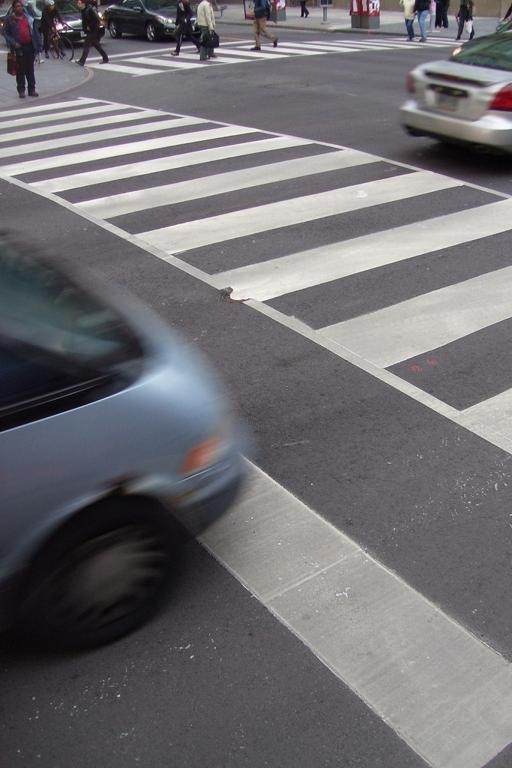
[17,86,26,97]
[27,86,39,96]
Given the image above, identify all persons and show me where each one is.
[435,0,450,32]
[454,0,476,41]
[194,0,219,61]
[399,0,416,41]
[2,0,43,98]
[76,0,109,65]
[169,0,201,55]
[24,0,43,66]
[250,0,279,52]
[41,0,65,58]
[298,0,311,19]
[412,0,434,43]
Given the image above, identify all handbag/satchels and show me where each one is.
[7,45,18,75]
[202,29,219,48]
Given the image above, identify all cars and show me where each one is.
[101,1,204,43]
[0,226,262,649]
[401,22,511,159]
[0,0,106,45]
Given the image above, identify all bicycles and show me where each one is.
[41,21,74,60]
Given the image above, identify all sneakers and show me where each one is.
[199,53,217,61]
[250,46,261,51]
[75,59,84,66]
[99,59,110,64]
[273,37,279,47]
[171,51,180,56]
[405,36,427,42]
